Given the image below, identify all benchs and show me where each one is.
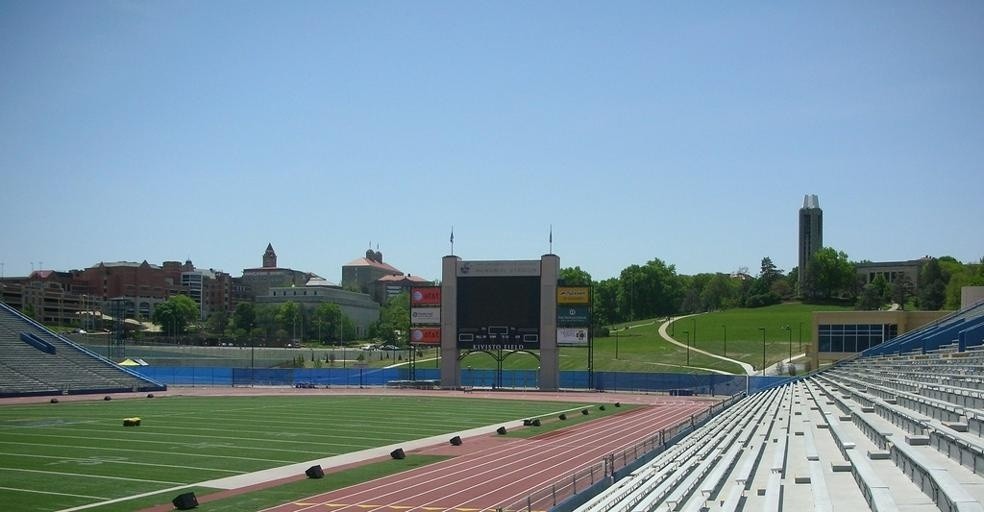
[0,305,157,393]
[573,342,979,512]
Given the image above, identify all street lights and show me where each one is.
[797,321,806,353]
[250,322,256,367]
[681,330,691,367]
[785,325,792,365]
[691,317,697,348]
[722,324,726,358]
[758,327,767,376]
[613,327,619,361]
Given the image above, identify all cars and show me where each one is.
[142,336,302,348]
[362,342,400,351]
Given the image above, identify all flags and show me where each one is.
[449,233,454,242]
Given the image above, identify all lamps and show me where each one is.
[171,491,199,511]
[305,465,325,480]
[390,448,406,460]
[446,412,568,447]
[50,393,154,404]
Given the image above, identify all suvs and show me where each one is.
[322,336,339,346]
